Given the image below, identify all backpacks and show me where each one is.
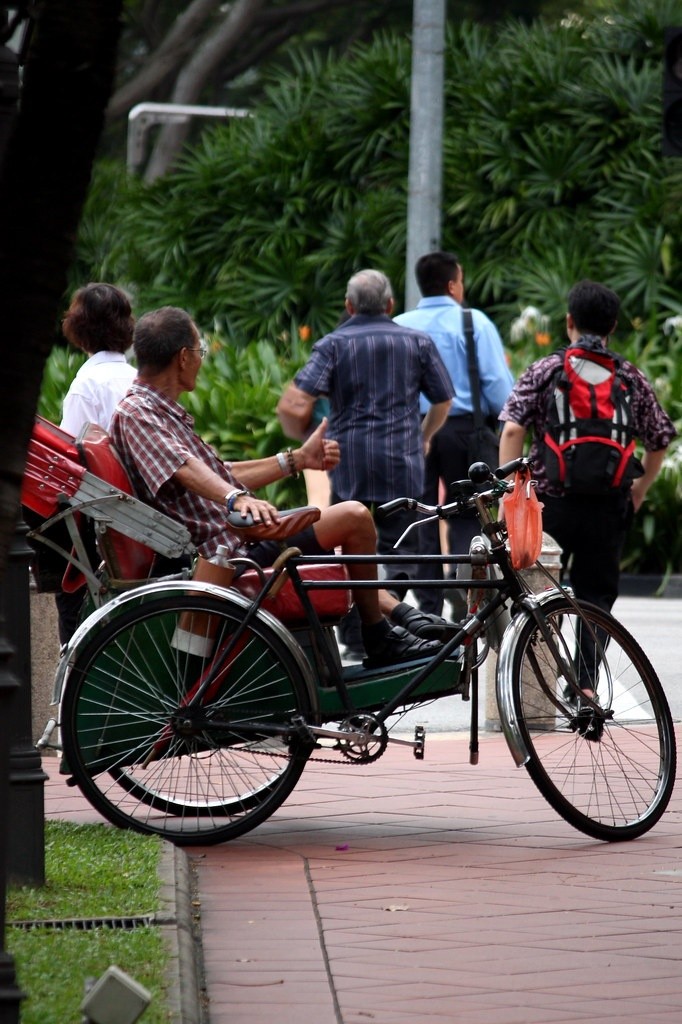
[533,348,646,499]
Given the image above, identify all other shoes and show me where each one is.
[443,585,468,622]
[569,687,597,706]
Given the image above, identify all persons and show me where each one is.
[108,306,463,669]
[393,252,514,624]
[280,270,457,662]
[499,281,678,706]
[58,281,138,651]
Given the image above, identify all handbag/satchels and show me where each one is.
[503,465,542,569]
[467,424,501,492]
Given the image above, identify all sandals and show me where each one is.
[400,611,462,644]
[362,626,441,667]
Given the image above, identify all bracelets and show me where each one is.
[225,489,241,508]
[276,453,286,473]
[228,491,252,511]
[287,448,300,478]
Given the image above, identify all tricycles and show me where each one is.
[24,413,677,846]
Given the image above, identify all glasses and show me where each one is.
[186,347,206,359]
